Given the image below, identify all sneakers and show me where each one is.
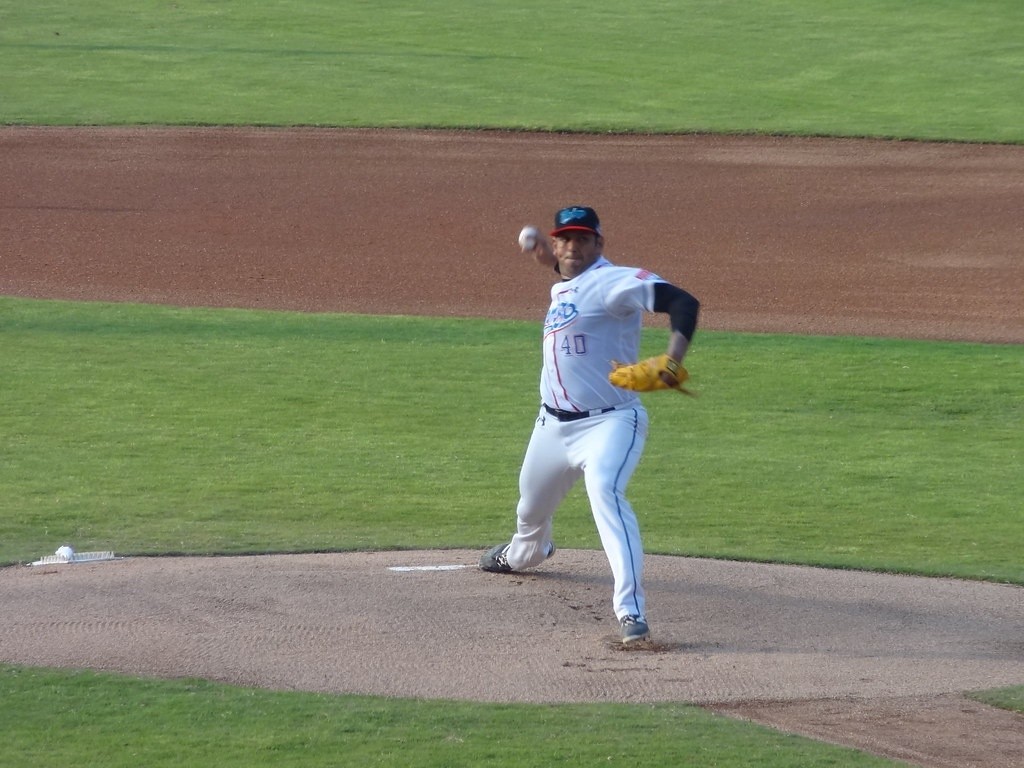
[620,614,649,643]
[478,541,555,572]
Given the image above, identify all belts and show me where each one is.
[543,403,615,422]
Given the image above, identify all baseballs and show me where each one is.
[518,226,537,249]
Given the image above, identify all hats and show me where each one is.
[549,205,600,236]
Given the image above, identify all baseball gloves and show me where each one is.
[608,353,690,392]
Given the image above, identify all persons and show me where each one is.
[479,206,700,645]
